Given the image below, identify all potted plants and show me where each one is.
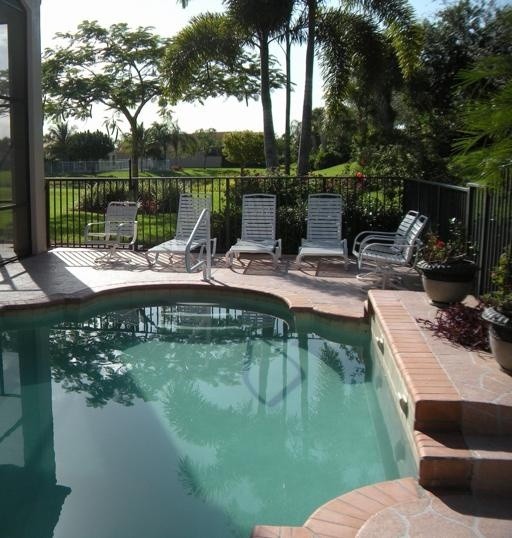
[481,248,510,378]
[406,216,480,306]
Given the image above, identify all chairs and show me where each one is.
[222,192,283,272]
[144,191,219,273]
[293,193,351,269]
[351,209,430,295]
[83,199,142,266]
